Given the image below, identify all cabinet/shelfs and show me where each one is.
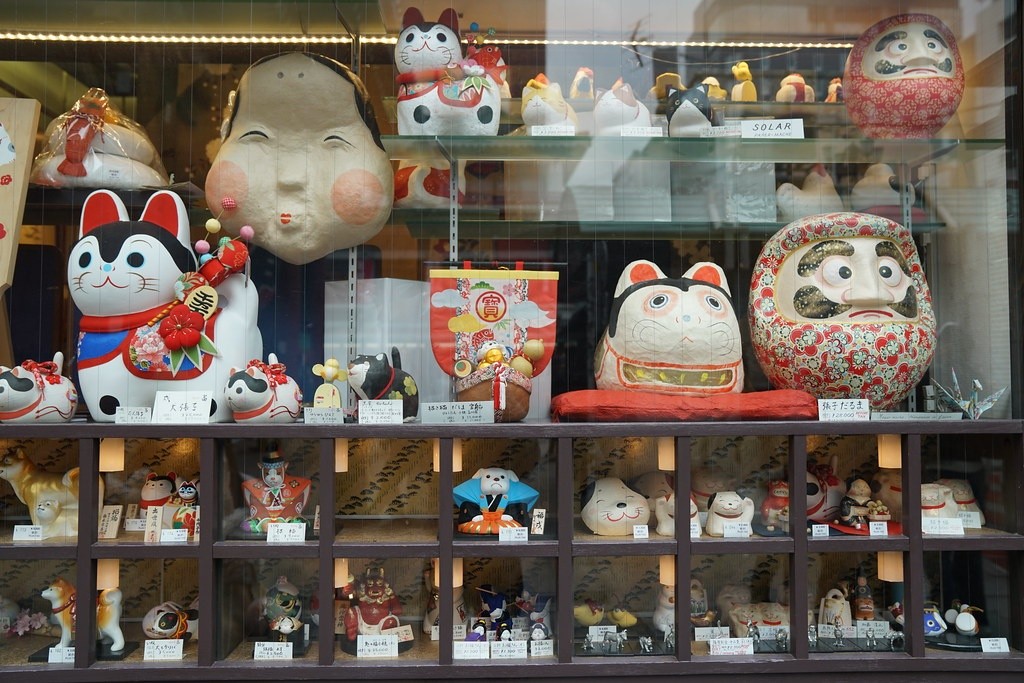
[0,416,1024,683]
